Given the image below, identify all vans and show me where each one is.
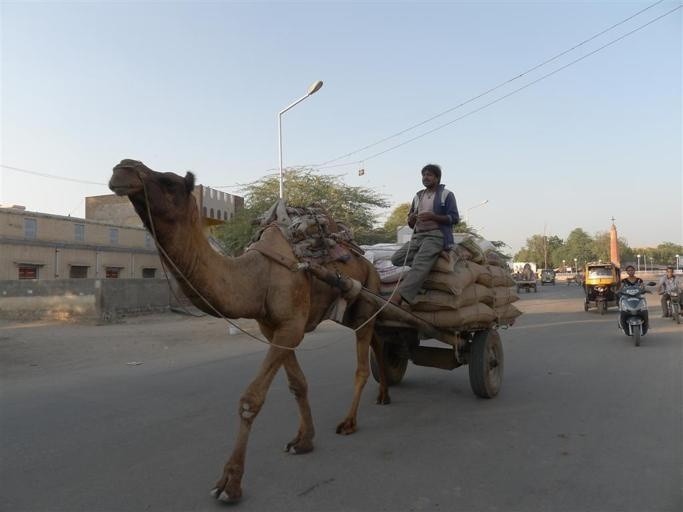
[540,269,555,286]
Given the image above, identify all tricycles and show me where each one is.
[514,270,537,294]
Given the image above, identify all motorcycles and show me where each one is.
[611,282,656,348]
[583,261,621,315]
[658,286,683,325]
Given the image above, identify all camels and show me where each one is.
[107,159,379,503]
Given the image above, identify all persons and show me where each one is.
[614,264,645,291]
[383,164,460,308]
[656,266,682,318]
[580,268,586,296]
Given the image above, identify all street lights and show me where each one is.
[636,254,640,270]
[649,257,653,270]
[276,79,322,202]
[675,254,679,270]
[573,258,577,274]
[466,200,488,226]
[562,260,565,276]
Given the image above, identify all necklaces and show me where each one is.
[426,190,434,199]
